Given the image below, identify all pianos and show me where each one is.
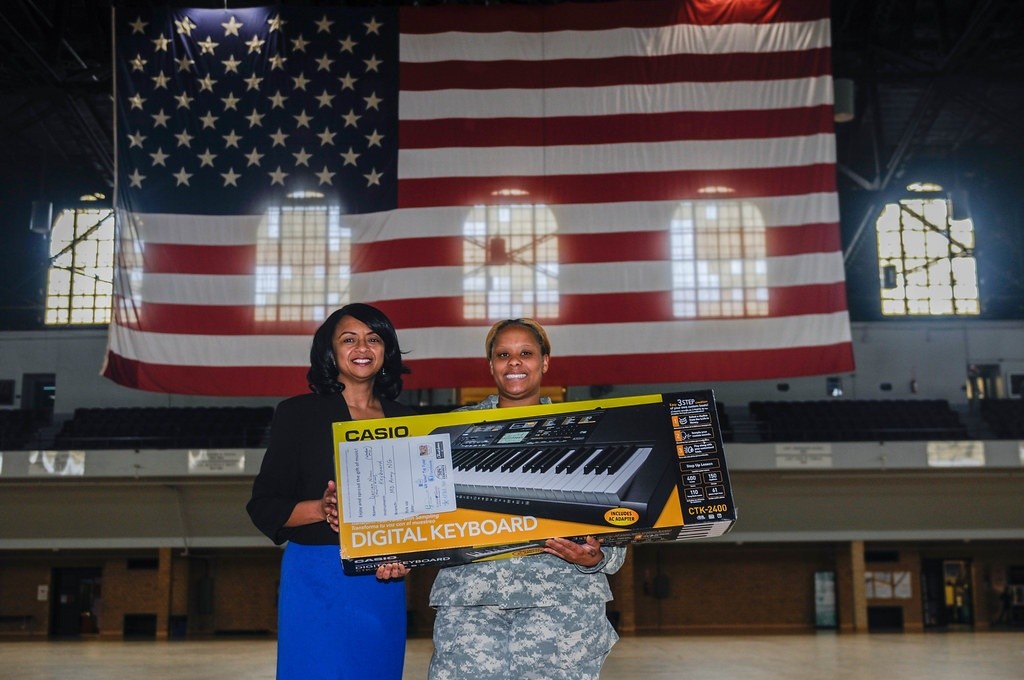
[426,403,679,529]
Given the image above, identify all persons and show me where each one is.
[427,318,627,680]
[246,303,419,680]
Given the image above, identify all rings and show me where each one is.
[326,514,330,523]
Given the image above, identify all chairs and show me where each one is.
[0,399,1024,450]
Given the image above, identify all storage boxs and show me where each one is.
[330,389,738,577]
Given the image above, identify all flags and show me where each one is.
[98,0,855,397]
